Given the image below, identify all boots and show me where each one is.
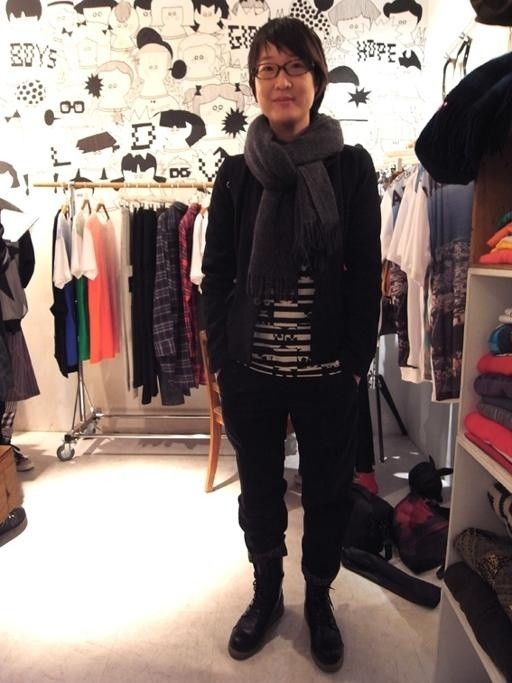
[305,584,344,673]
[227,569,284,661]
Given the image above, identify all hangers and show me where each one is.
[54,181,214,237]
[377,163,475,197]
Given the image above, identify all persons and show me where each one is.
[195,15,381,673]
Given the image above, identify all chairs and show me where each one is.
[199,330,294,492]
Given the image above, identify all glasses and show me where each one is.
[254,58,315,81]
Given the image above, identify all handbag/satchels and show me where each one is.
[344,482,451,576]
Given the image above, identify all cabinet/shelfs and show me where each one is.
[433,152,512,683]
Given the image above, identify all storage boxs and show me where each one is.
[0,445,23,524]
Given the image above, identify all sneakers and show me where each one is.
[0,508,28,546]
[14,450,34,471]
